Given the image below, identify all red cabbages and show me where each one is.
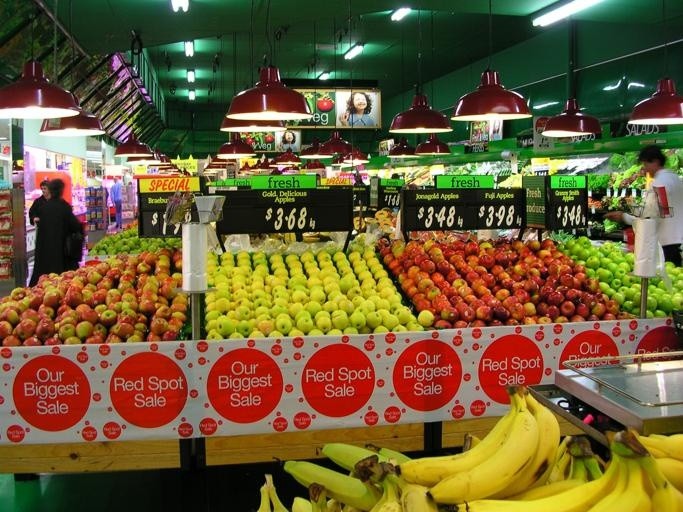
[620,167,648,188]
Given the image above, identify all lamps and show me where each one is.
[182,40,195,58]
[450,1,532,121]
[389,5,412,24]
[539,19,602,137]
[170,1,191,14]
[0,0,106,138]
[628,0,683,125]
[185,69,197,85]
[187,88,196,103]
[531,0,608,28]
[316,69,330,82]
[342,41,366,63]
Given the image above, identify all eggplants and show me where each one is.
[665,148,676,155]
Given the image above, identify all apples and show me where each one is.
[264,134,274,142]
[0,225,683,346]
[245,137,254,144]
[317,97,334,111]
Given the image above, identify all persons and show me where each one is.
[28,180,53,227]
[338,92,373,127]
[488,120,502,141]
[109,176,122,233]
[276,130,296,151]
[29,178,80,288]
[601,143,681,267]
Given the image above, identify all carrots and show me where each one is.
[588,197,603,208]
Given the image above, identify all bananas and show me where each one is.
[257,384,683,512]
